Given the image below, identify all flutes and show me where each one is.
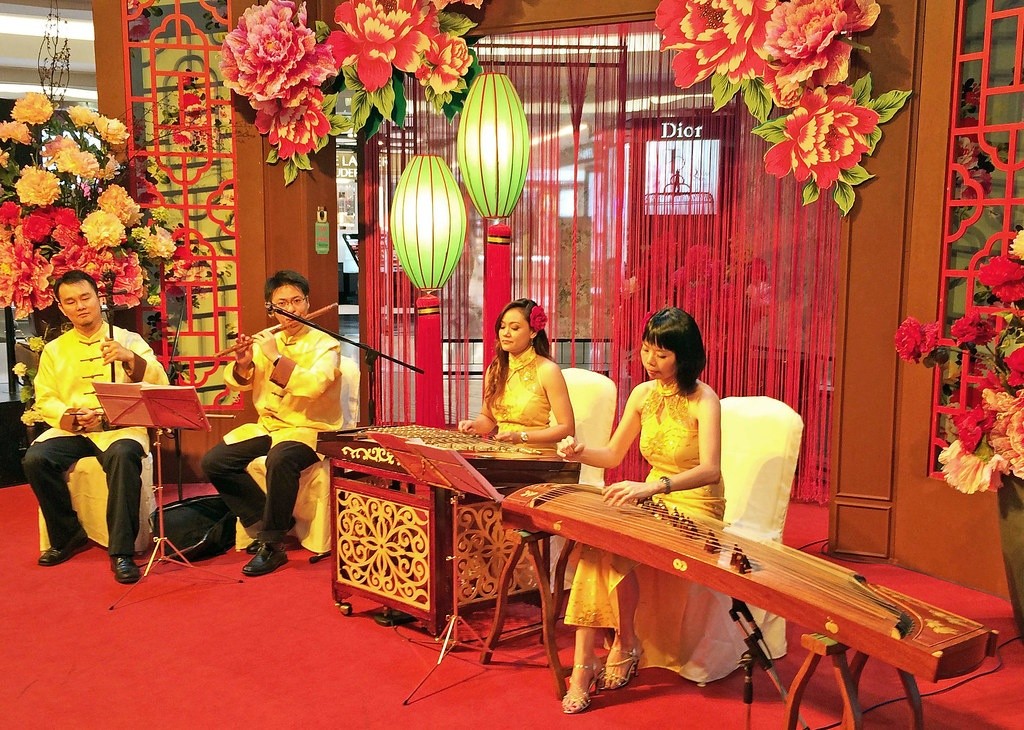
[213,302,339,359]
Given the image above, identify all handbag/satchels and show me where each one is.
[149,494,236,561]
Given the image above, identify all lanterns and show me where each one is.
[390,154,467,429]
[457,72,531,439]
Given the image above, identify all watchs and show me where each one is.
[659,476,671,494]
[520,431,528,442]
[272,355,282,366]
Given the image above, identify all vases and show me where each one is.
[998,470,1024,641]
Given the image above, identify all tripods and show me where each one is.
[367,433,551,705]
[92,382,244,611]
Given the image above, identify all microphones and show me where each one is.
[731,597,763,639]
[265,302,274,318]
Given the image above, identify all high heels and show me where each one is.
[596,638,644,689]
[561,666,606,714]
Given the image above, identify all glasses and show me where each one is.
[273,296,309,309]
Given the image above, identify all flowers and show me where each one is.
[895,75,1024,498]
[654,0,912,217]
[0,0,183,426]
[219,0,486,187]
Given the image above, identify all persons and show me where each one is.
[200,270,344,575]
[557,307,728,713]
[458,298,575,443]
[21,270,170,584]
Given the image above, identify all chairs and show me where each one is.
[39,452,157,555]
[517,368,616,592]
[235,354,361,566]
[669,393,806,691]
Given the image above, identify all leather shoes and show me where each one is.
[39,529,89,565]
[246,539,264,554]
[243,542,288,575]
[110,554,142,582]
